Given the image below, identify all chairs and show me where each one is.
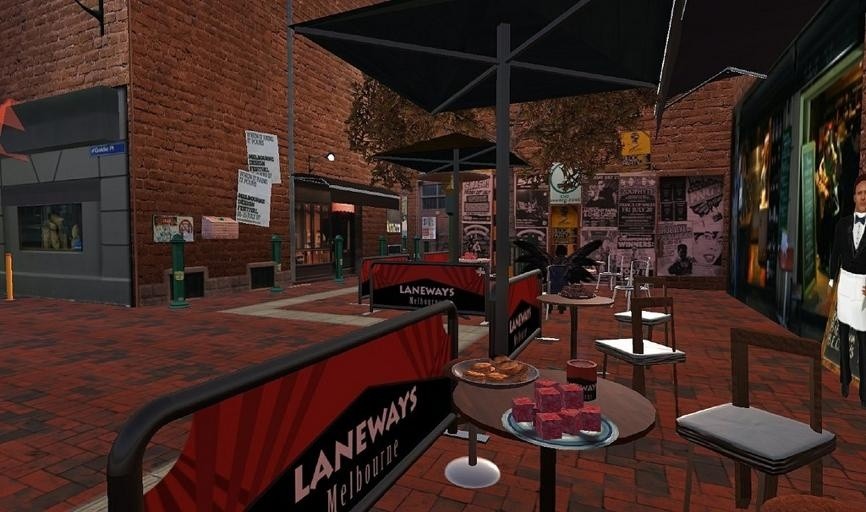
[675,326,836,510]
[595,252,686,398]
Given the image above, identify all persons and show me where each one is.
[694,231,724,278]
[827,173,865,407]
[667,244,692,279]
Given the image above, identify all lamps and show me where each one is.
[307,152,335,175]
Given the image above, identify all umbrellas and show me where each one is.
[288,0,674,357]
[372,137,532,272]
